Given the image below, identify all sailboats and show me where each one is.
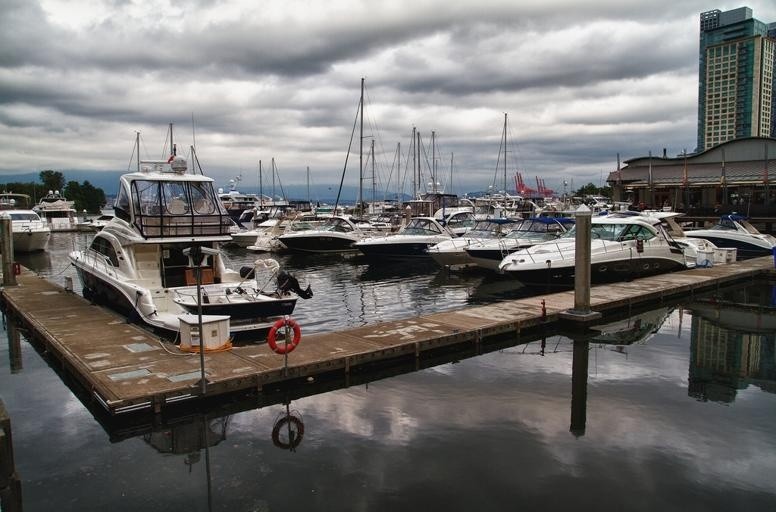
[216,76,534,270]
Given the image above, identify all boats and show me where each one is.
[683,211,776,257]
[137,411,228,473]
[0,183,122,253]
[425,217,523,273]
[69,122,312,346]
[462,210,578,277]
[498,211,717,290]
[499,304,684,383]
